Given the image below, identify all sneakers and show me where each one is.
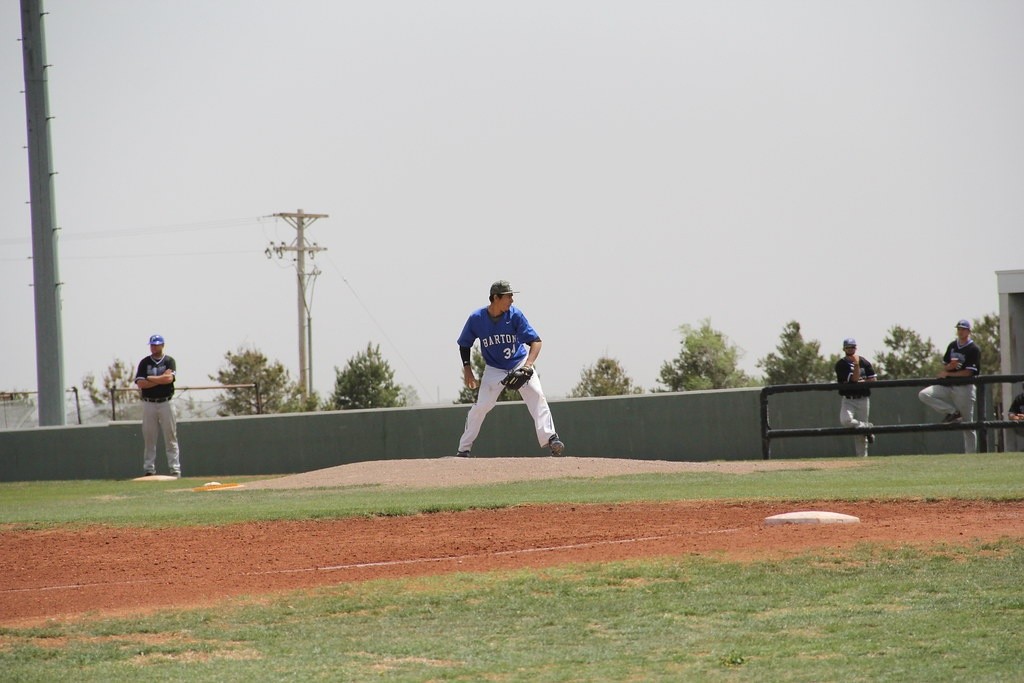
[456,449,470,457]
[548,433,564,457]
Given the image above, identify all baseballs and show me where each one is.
[468,381,476,389]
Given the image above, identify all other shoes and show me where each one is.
[942,411,962,425]
[144,470,156,475]
[867,423,875,444]
[172,471,181,476]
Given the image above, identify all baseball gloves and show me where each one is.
[501,366,533,390]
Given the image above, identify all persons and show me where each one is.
[135,335,182,476]
[1008,383,1024,438]
[456,280,565,456]
[918,319,980,454]
[836,338,877,458]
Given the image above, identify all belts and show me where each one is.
[145,397,171,402]
[845,394,868,399]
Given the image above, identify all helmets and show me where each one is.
[148,335,164,345]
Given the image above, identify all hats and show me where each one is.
[956,320,970,330]
[844,338,856,346]
[490,280,520,294]
